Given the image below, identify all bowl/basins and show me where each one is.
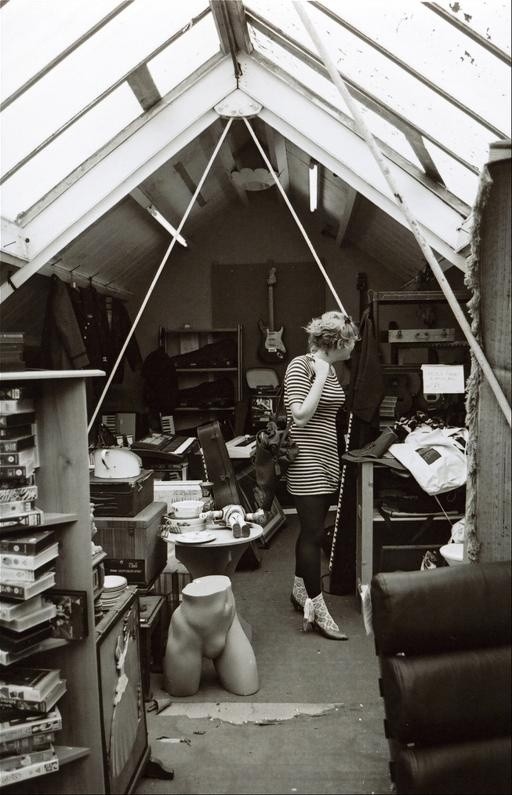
[162,499,210,533]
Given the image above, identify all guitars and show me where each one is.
[256,267,286,364]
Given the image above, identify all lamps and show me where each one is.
[147,206,190,248]
[309,158,324,213]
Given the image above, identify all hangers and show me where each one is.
[41,272,122,316]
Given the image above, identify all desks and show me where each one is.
[159,520,265,642]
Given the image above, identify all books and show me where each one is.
[1,321,69,786]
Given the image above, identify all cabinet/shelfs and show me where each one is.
[355,288,472,615]
[158,324,244,436]
[92,502,168,591]
[0,370,110,795]
[93,585,174,795]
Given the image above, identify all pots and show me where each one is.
[93,448,142,478]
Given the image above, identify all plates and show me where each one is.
[174,532,217,544]
[99,574,127,608]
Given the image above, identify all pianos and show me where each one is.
[160,415,176,435]
[226,434,256,458]
[102,414,136,442]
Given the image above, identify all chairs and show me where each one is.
[358,557,512,794]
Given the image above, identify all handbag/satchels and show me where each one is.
[390,426,469,495]
[254,425,299,486]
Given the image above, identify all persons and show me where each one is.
[197,504,265,538]
[282,310,362,640]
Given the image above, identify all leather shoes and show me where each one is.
[291,576,309,612]
[303,596,347,640]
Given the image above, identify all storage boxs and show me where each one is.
[89,467,156,516]
